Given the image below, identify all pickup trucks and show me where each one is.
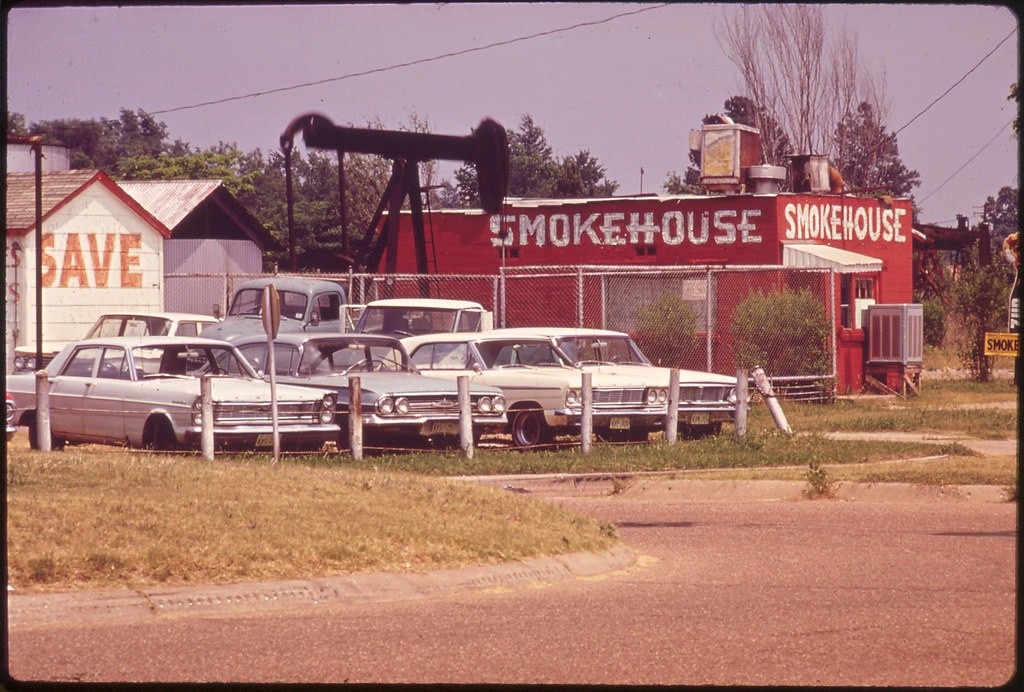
[184,263,413,371]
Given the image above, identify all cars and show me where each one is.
[5,299,752,456]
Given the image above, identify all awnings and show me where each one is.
[782,244,882,273]
